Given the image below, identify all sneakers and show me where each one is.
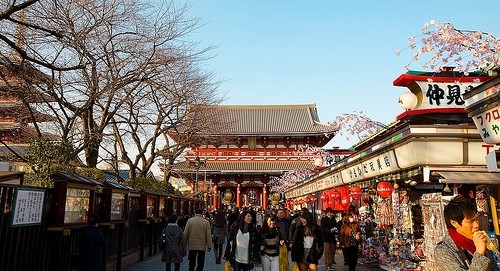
[326,265,335,271]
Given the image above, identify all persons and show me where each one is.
[258,214,285,271]
[434,193,500,271]
[290,211,324,271]
[289,208,311,247]
[223,210,262,271]
[211,211,229,264]
[276,208,291,253]
[320,207,338,271]
[340,213,362,271]
[160,214,186,271]
[228,205,278,229]
[328,211,338,264]
[338,213,352,264]
[184,208,214,271]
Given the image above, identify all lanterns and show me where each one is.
[330,189,341,201]
[299,199,304,205]
[321,192,329,202]
[294,200,299,205]
[376,182,393,198]
[304,197,311,203]
[310,195,317,202]
[398,92,418,109]
[290,202,294,206]
[350,186,362,199]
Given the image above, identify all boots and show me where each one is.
[214,249,221,264]
[218,247,223,262]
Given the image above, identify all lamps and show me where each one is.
[398,92,418,111]
